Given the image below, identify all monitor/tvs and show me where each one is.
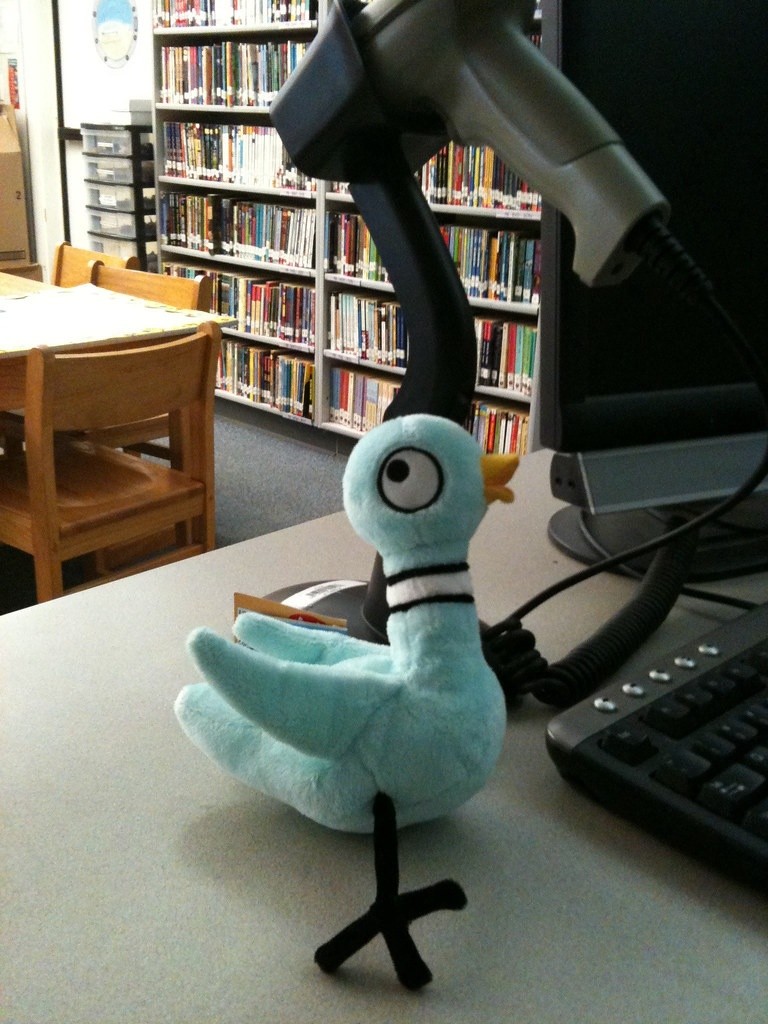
[535,0,768,581]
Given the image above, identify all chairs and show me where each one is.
[0,239,238,605]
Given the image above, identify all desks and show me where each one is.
[0,447,768,1024]
[0,270,237,361]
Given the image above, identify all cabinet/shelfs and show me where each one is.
[315,0,564,455]
[79,123,158,274]
[150,0,322,426]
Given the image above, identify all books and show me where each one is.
[160,261,316,347]
[437,222,541,305]
[162,118,354,194]
[475,316,536,394]
[415,140,543,211]
[324,210,390,285]
[327,367,401,434]
[154,0,320,30]
[330,295,410,368]
[468,400,529,457]
[160,189,315,270]
[214,336,316,419]
[161,40,314,106]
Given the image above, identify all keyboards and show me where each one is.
[544,599,767,893]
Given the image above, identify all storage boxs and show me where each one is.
[0,104,31,270]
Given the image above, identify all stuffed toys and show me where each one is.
[176,414,518,990]
